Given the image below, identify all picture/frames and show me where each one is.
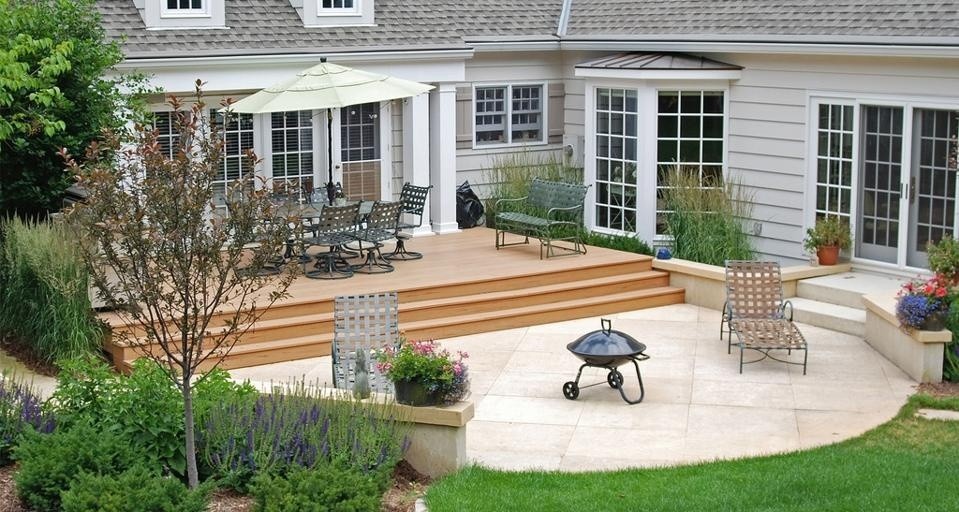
[606,185,636,231]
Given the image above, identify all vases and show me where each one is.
[376,330,471,405]
[895,273,959,336]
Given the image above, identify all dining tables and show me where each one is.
[803,213,853,265]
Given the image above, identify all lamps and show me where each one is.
[263,182,434,279]
[720,260,808,375]
[331,289,398,393]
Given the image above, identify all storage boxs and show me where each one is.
[495,176,592,259]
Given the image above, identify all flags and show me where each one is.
[394,377,429,404]
[922,309,949,331]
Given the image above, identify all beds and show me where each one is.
[217,57,437,250]
